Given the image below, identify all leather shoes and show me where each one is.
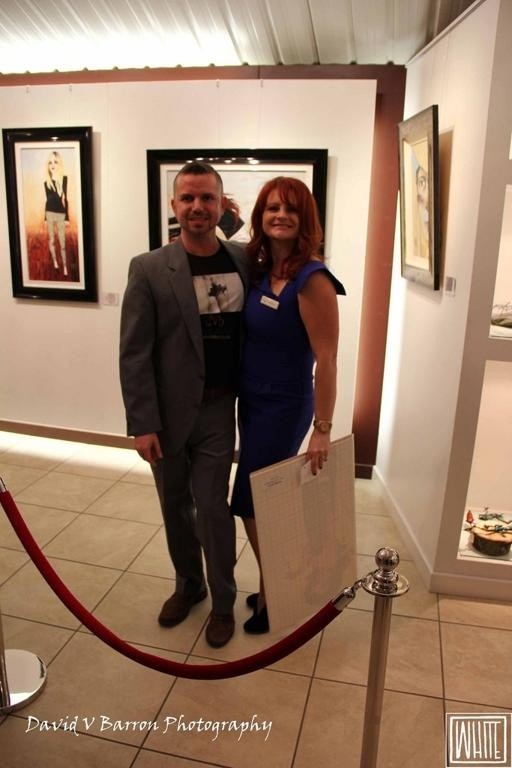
[205,599,235,649]
[157,583,209,628]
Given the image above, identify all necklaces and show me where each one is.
[270,270,289,282]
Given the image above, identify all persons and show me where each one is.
[228,174,350,633]
[118,161,250,650]
[40,149,70,280]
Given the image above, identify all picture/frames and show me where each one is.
[2,125,97,302]
[147,148,328,256]
[400,103,443,289]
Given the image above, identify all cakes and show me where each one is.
[464,506,512,556]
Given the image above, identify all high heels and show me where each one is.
[243,592,269,635]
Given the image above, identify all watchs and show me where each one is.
[312,417,334,434]
